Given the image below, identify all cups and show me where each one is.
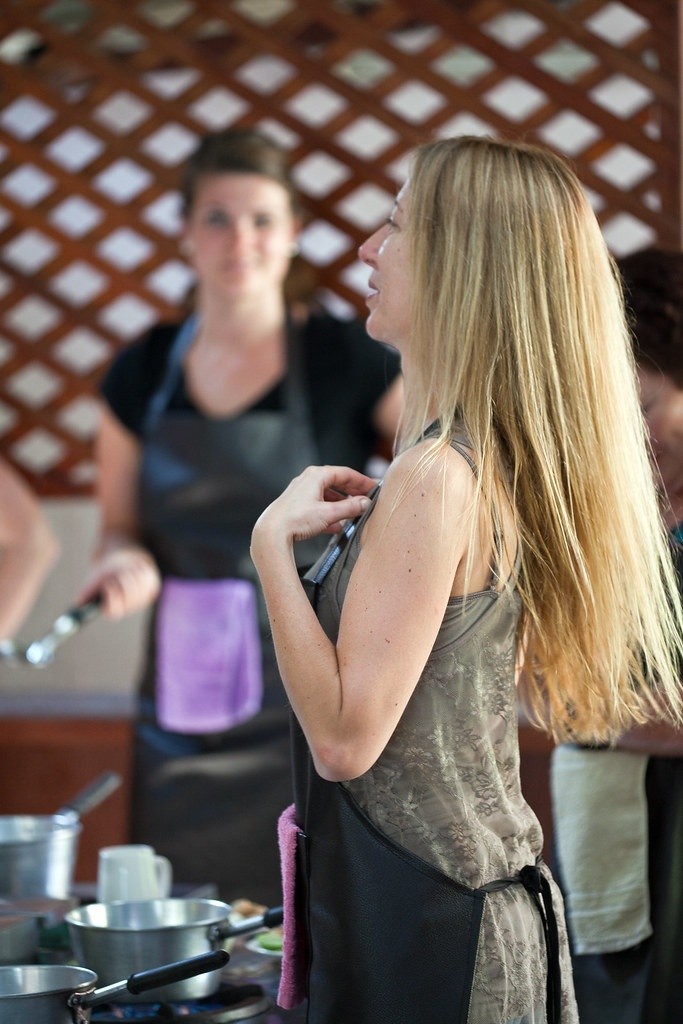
[95,843,174,913]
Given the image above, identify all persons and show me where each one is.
[0,451,57,645]
[249,133,683,1023]
[77,129,408,906]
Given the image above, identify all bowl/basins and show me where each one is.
[63,895,290,1007]
[0,949,229,1023]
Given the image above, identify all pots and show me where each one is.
[0,769,124,910]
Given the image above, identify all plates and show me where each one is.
[245,935,284,958]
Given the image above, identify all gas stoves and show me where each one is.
[0,913,278,1024]
